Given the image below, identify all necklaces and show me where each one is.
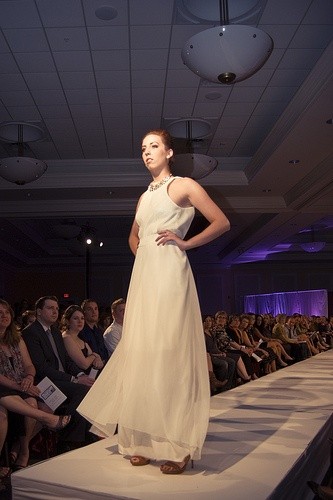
[148,173,173,192]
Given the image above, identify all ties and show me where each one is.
[46,328,65,374]
[236,329,242,344]
[93,325,103,360]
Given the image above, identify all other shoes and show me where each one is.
[318,345,332,353]
[281,362,288,367]
[0,452,29,481]
[285,357,295,361]
[307,480,333,500]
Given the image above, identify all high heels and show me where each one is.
[130,456,151,466]
[160,454,193,474]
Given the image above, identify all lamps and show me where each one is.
[300,225,326,253]
[49,224,82,240]
[0,124,47,185]
[181,0,274,85]
[168,121,219,179]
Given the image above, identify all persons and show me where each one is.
[308,479,333,500]
[0,295,333,479]
[79,134,232,474]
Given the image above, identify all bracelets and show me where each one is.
[25,374,35,378]
[89,353,96,358]
[240,347,242,351]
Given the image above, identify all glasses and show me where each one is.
[217,316,227,320]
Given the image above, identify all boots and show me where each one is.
[209,373,228,391]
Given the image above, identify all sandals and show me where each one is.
[42,415,71,432]
